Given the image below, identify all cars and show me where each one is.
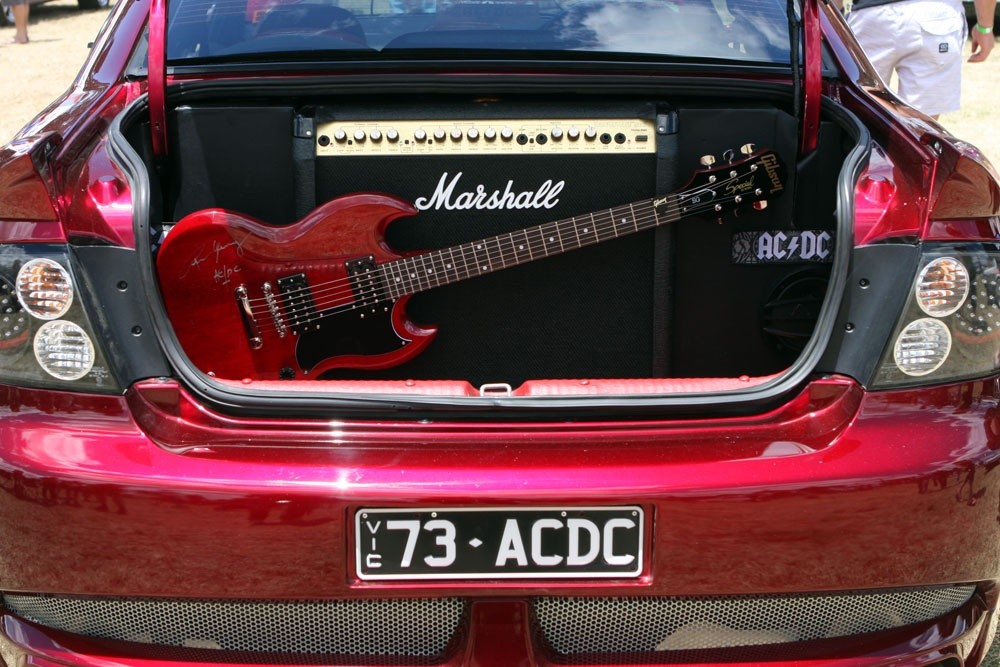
[4,2,997,666]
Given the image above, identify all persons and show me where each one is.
[831,0,996,120]
[1,0,29,45]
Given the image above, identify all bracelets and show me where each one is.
[976,22,994,34]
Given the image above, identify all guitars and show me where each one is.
[154,140,791,380]
